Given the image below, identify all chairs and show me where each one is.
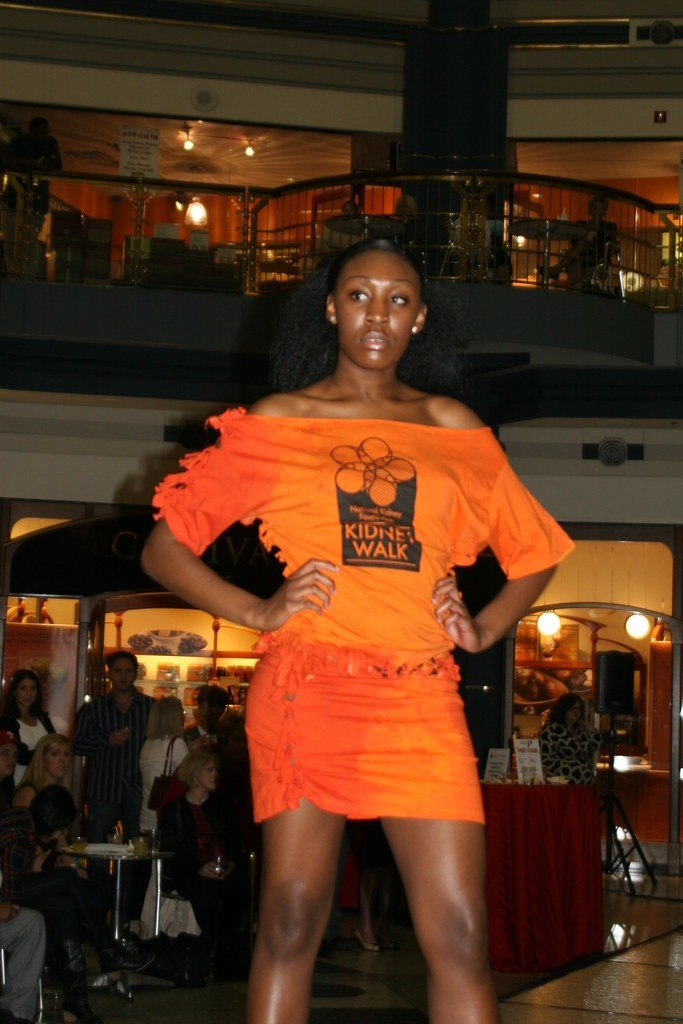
[558,240,627,302]
[139,832,204,982]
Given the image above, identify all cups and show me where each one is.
[133,836,149,854]
[210,853,226,875]
[72,836,86,853]
[107,824,124,844]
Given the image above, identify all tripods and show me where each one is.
[599,715,657,898]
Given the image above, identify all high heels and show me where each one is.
[101,952,156,982]
[62,992,104,1024]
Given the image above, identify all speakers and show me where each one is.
[594,651,636,715]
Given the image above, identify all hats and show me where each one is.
[0,729,28,753]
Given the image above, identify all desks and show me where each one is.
[53,843,174,1000]
[507,219,587,290]
[480,782,604,972]
[326,213,407,241]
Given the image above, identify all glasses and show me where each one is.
[569,707,582,712]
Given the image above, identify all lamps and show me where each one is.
[183,126,194,150]
[537,610,561,636]
[624,614,650,640]
[184,197,207,225]
[244,140,254,156]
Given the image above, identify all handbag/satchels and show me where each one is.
[147,735,182,810]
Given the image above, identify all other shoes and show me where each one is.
[353,929,380,952]
[377,936,399,949]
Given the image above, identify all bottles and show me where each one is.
[149,829,160,854]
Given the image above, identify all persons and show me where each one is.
[536,197,619,289]
[538,693,603,784]
[71,653,263,986]
[143,240,576,1024]
[343,201,357,215]
[349,821,397,951]
[0,669,156,1024]
[393,196,416,221]
[0,117,62,280]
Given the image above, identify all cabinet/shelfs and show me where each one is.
[86,646,261,726]
